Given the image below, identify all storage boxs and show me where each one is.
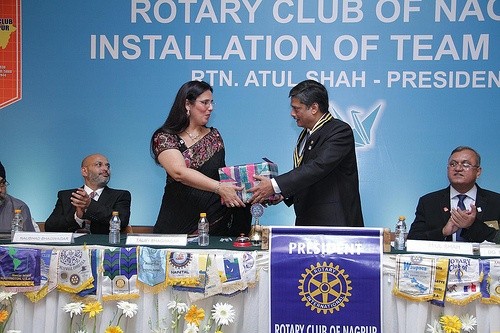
[219,158,278,205]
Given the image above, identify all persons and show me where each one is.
[149,81,246,235]
[0,161,36,232]
[248,80,365,227]
[407,146,500,243]
[44,153,131,234]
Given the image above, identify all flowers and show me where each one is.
[106,300,138,333]
[148,293,236,333]
[63,301,104,333]
[0,291,21,333]
[426,314,478,333]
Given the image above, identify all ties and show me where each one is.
[84,192,96,230]
[456,195,468,242]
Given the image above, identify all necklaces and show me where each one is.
[185,129,203,139]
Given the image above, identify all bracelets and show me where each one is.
[215,182,222,194]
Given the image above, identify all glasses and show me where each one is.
[448,162,479,171]
[190,100,217,108]
[0,180,9,186]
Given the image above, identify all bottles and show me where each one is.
[394,215,407,251]
[108,212,121,245]
[10,209,23,242]
[197,213,209,246]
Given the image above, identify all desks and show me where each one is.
[0,234,500,333]
